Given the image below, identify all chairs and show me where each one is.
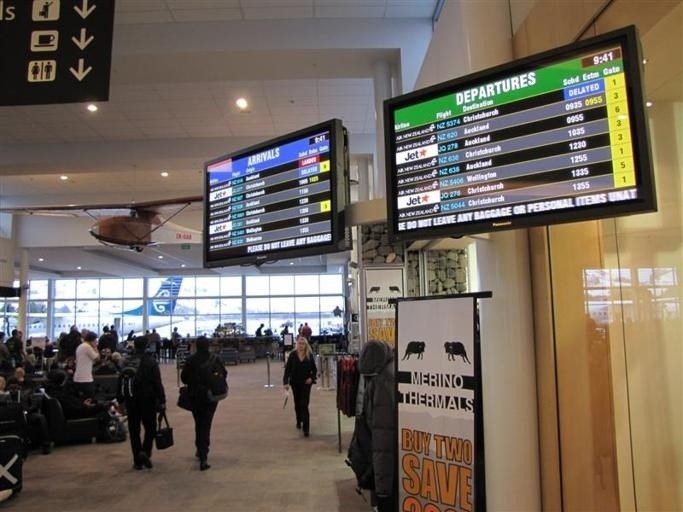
[175,344,257,368]
[0,335,175,447]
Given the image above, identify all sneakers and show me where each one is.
[196,449,211,470]
[132,453,153,470]
[295,421,310,438]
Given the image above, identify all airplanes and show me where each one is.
[0,276,197,347]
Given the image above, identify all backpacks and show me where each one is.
[198,364,228,402]
[117,356,148,402]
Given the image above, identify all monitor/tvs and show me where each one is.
[383,25,658,245]
[203,118,352,269]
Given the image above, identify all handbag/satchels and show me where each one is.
[154,414,175,450]
[176,385,200,413]
[282,390,289,410]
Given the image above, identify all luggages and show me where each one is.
[0,432,25,506]
